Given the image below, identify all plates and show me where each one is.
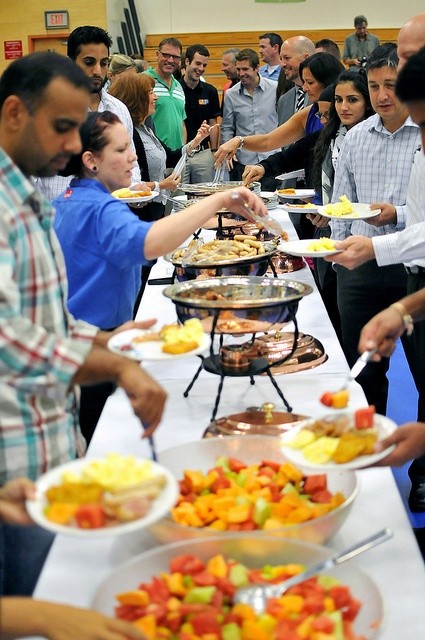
[275,167,306,182]
[274,188,317,199]
[279,412,400,471]
[25,453,182,539]
[277,239,343,258]
[112,186,158,208]
[317,203,382,221]
[107,324,212,360]
[276,204,318,213]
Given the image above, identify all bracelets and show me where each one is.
[389,302,415,339]
[234,135,245,151]
[188,140,201,153]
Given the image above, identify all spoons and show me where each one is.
[235,527,394,617]
[321,348,378,408]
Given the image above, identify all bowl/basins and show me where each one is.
[164,241,279,282]
[162,275,312,334]
[154,434,358,549]
[92,536,385,640]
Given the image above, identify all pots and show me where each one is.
[184,181,262,202]
[184,198,271,230]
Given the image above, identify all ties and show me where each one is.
[295,84,307,113]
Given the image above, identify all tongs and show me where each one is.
[229,213,232,214]
[211,157,230,183]
[231,192,283,237]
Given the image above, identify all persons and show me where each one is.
[327,42,422,515]
[241,67,332,238]
[344,15,380,73]
[220,49,278,192]
[50,110,268,443]
[33,26,157,219]
[315,38,341,62]
[213,52,348,170]
[303,66,376,322]
[141,37,187,177]
[0,477,150,640]
[359,49,425,469]
[277,35,317,234]
[0,49,170,597]
[106,54,137,87]
[179,44,221,184]
[323,12,425,297]
[107,73,221,221]
[221,47,241,118]
[257,32,284,81]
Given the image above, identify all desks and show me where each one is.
[27,191,424,640]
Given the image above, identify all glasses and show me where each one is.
[160,51,182,61]
